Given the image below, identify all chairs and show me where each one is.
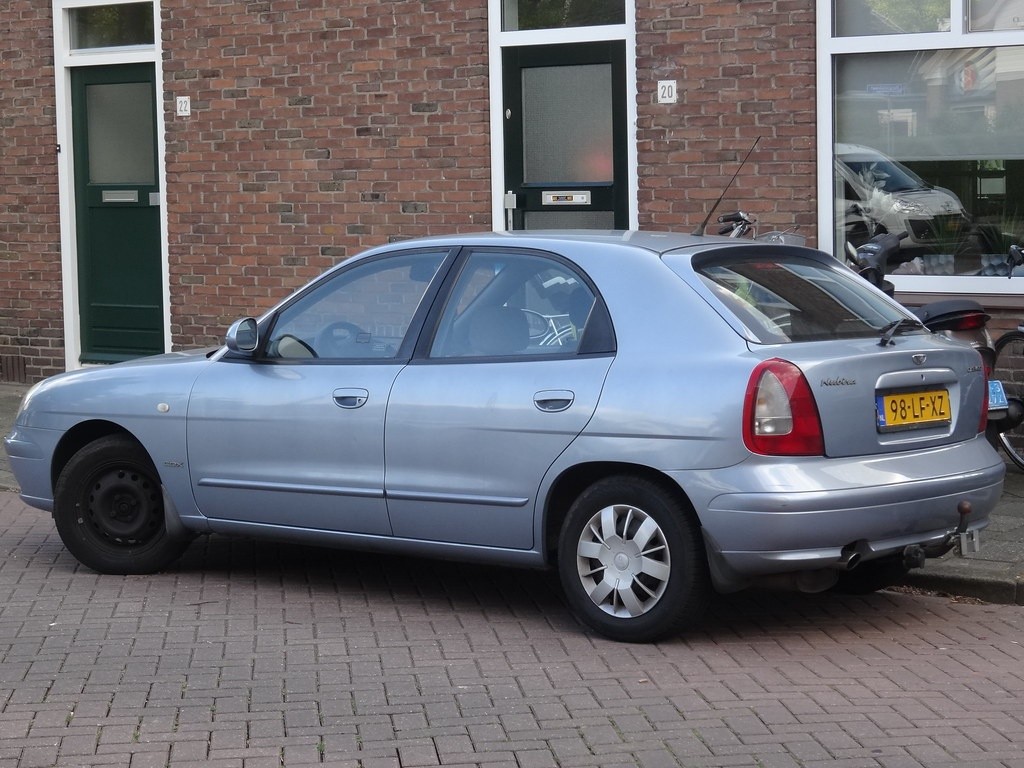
[457,304,529,357]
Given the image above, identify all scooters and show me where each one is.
[844,231,1023,456]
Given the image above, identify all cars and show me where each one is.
[834,45,999,136]
[5,230,1009,642]
[833,140,984,279]
